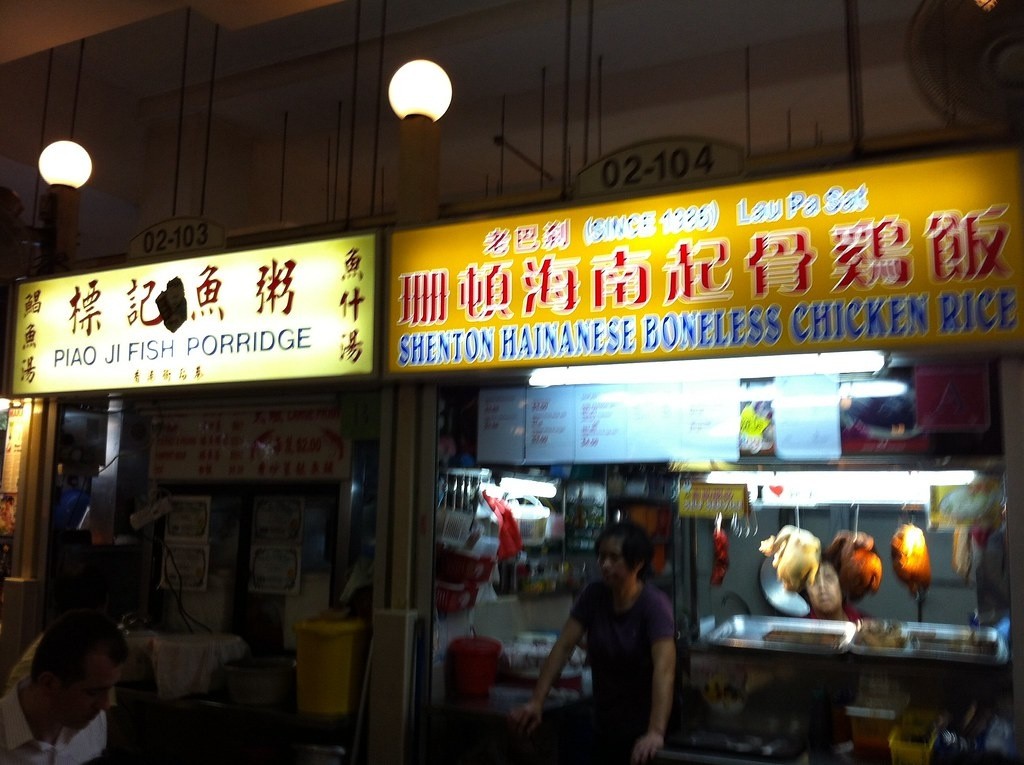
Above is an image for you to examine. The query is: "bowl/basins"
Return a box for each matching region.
[224,656,297,705]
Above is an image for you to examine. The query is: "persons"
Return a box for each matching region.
[798,554,874,623]
[508,520,675,765]
[0,564,131,765]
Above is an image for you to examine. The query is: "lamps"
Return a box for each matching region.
[38,140,93,191]
[387,59,453,122]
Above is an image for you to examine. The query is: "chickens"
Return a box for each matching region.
[759,524,933,623]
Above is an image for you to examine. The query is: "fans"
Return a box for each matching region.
[902,0,1024,139]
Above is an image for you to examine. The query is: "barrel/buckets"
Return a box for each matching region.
[450,625,502,693]
[292,616,365,716]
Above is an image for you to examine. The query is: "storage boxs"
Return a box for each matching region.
[887,724,938,765]
[903,708,944,729]
[849,717,902,759]
